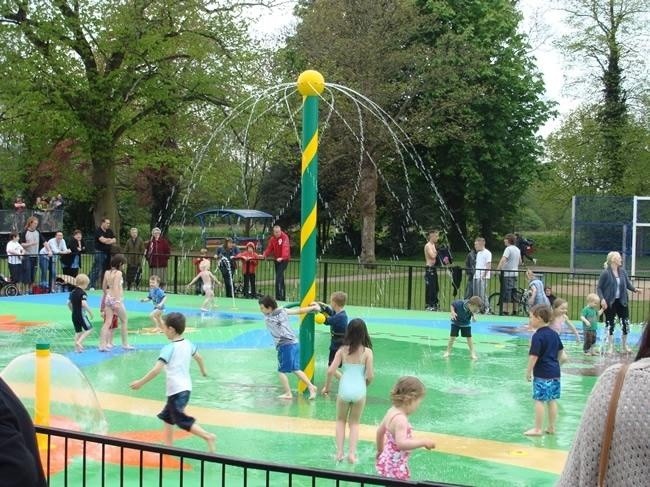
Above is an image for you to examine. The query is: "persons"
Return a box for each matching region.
[184,226,291,313]
[441,295,483,361]
[320,318,374,464]
[523,304,567,436]
[1,378,49,486]
[465,251,476,300]
[258,295,321,400]
[473,237,493,316]
[127,312,216,454]
[5,194,172,353]
[308,291,349,380]
[554,317,649,487]
[374,376,436,486]
[515,235,537,266]
[524,251,643,356]
[424,229,449,311]
[492,234,523,315]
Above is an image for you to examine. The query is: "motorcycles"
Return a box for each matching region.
[0,273,20,297]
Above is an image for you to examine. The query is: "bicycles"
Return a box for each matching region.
[232,276,264,300]
[489,285,530,316]
